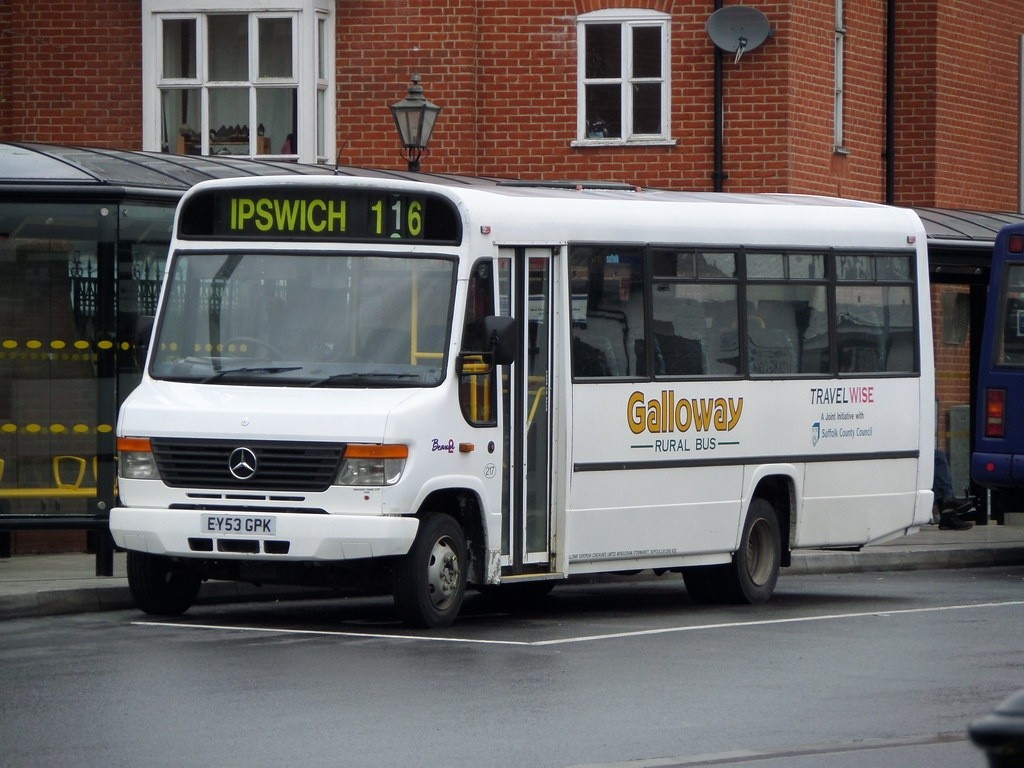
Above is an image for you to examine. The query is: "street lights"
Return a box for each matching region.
[386,70,444,175]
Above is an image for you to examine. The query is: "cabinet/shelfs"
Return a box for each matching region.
[177,134,272,156]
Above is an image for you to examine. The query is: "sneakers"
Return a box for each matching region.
[939,516,973,530]
[941,498,973,515]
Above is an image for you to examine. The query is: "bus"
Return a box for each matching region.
[109,174,940,632]
[969,224,1024,494]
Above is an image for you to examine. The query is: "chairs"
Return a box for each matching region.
[367,298,913,377]
